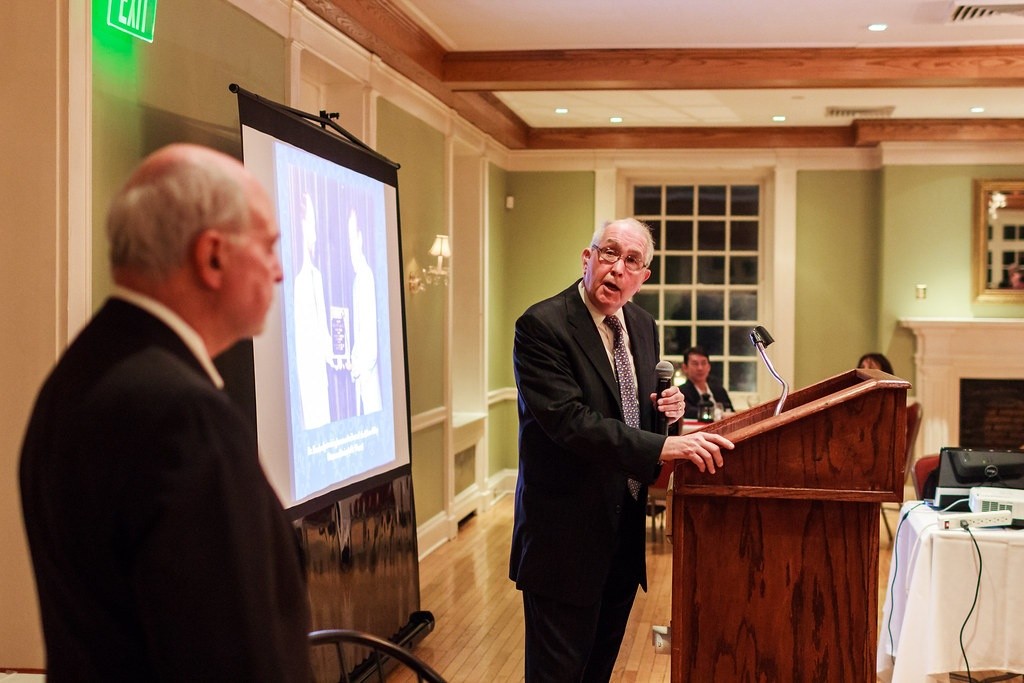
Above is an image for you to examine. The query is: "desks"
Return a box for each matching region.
[882,500,1024,683]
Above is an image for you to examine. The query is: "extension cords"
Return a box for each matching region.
[937,510,1013,530]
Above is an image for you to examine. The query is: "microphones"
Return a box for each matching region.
[655,360,674,435]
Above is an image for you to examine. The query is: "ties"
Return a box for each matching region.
[602,316,639,500]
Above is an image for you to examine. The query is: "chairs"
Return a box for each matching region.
[880,402,924,542]
[645,461,675,544]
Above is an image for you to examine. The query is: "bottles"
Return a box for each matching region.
[698,394,714,422]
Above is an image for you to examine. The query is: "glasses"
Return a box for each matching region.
[593,244,646,271]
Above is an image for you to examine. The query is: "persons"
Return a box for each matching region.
[288,189,385,428]
[507,218,735,683]
[17,138,313,683]
[672,348,736,430]
[857,352,896,376]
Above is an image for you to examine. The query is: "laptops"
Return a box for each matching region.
[924,447,1024,515]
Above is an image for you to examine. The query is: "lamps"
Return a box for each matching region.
[410,234,454,294]
